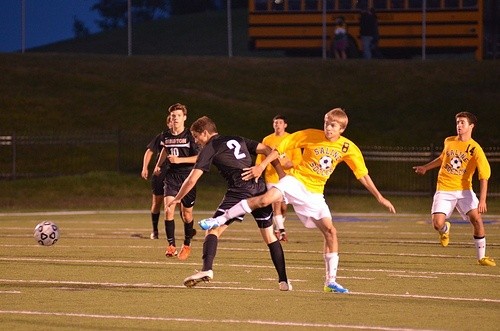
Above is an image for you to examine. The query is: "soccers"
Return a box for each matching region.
[33,221,59,246]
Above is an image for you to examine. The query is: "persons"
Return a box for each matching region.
[140,102,203,262]
[413,112,496,267]
[335,17,351,59]
[257,115,303,241]
[164,116,294,291]
[356,2,380,60]
[199,108,397,294]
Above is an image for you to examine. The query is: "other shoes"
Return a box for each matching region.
[150,232,158,239]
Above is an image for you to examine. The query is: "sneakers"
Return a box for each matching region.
[478,257,495,266]
[274,232,280,238]
[440,222,451,247]
[183,270,213,288]
[177,243,191,259]
[280,232,288,241]
[279,281,291,290]
[198,217,219,230]
[165,246,177,256]
[324,282,349,292]
